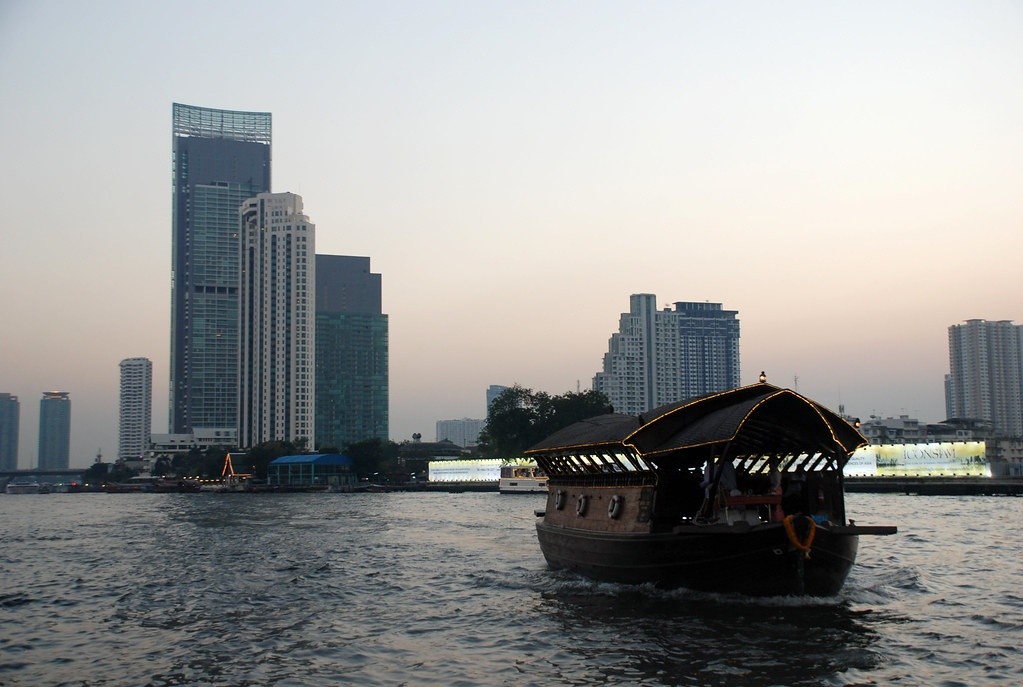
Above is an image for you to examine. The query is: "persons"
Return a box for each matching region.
[697,450,764,524]
[780,463,841,519]
[766,458,784,524]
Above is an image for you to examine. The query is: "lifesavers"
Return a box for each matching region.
[555,489,564,509]
[607,494,622,519]
[576,493,585,515]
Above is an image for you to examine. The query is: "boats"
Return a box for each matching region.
[524,370,898,605]
